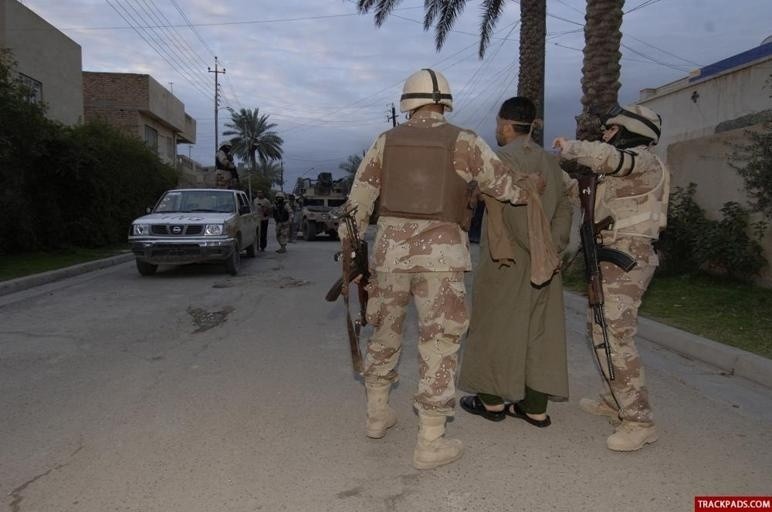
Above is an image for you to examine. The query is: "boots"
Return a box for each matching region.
[364,382,397,439]
[413,403,464,470]
[275,244,287,254]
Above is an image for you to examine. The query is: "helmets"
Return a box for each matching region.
[275,192,285,199]
[399,68,453,115]
[604,102,661,146]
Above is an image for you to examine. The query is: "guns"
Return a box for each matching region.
[578,173,637,381]
[326,199,400,388]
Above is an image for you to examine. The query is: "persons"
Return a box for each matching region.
[271,192,294,254]
[548,102,672,451]
[453,94,585,429]
[286,194,301,244]
[333,67,550,471]
[252,189,273,253]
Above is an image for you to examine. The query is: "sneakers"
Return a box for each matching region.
[607,419,658,452]
[579,397,617,419]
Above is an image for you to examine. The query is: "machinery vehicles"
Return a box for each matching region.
[294,169,347,241]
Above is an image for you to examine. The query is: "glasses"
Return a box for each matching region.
[605,105,622,118]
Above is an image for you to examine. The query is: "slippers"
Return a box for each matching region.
[505,401,551,428]
[459,395,505,422]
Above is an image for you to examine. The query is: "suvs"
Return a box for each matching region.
[119,185,263,276]
